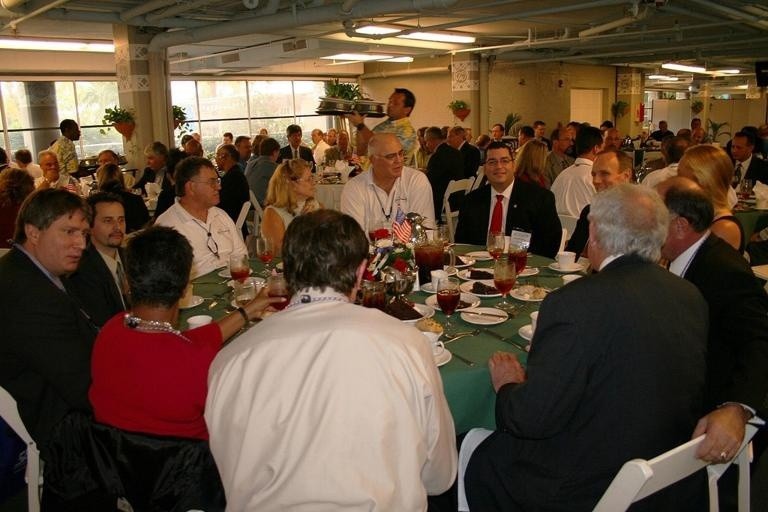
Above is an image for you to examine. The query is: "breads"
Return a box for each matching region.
[415,319,443,333]
[533,288,546,299]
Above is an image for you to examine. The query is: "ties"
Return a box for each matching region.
[115,261,134,314]
[294,149,297,160]
[735,163,742,182]
[488,194,505,241]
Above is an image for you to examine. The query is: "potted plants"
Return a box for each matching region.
[706,118,731,148]
[691,100,704,114]
[171,105,193,139]
[322,82,363,120]
[99,104,141,156]
[446,100,471,126]
[612,100,630,119]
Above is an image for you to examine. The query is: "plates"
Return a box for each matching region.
[392,248,547,366]
[218,267,253,278]
[319,95,386,105]
[178,295,206,311]
[732,194,758,212]
[548,263,586,273]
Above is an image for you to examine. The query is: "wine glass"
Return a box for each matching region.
[485,230,532,310]
[283,158,368,184]
[64,183,159,209]
[231,235,290,322]
[731,176,752,201]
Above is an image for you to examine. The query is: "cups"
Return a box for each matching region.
[187,314,213,328]
[179,282,193,303]
[415,222,457,288]
[358,283,387,311]
[557,249,576,269]
[561,275,582,287]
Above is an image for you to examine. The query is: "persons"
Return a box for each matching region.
[1,89,767,511]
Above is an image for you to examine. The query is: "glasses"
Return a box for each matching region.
[485,157,513,166]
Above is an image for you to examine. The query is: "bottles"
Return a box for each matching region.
[623,136,631,146]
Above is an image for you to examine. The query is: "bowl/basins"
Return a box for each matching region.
[79,159,97,167]
